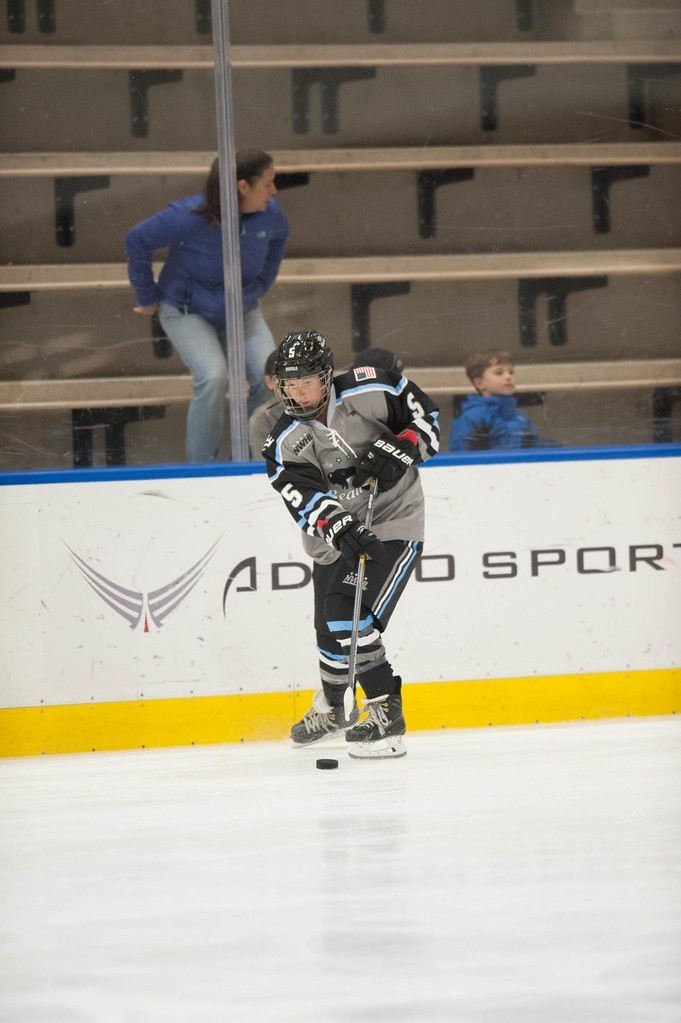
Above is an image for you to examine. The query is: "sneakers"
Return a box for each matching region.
[289,690,359,748]
[344,675,406,759]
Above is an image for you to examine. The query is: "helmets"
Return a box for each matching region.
[271,331,335,421]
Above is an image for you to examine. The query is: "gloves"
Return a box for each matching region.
[322,511,386,571]
[352,432,420,493]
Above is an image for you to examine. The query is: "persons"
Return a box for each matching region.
[126,147,290,463]
[262,330,440,757]
[448,346,559,451]
[250,349,285,462]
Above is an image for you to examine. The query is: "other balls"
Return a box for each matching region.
[316,759,339,769]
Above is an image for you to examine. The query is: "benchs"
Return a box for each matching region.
[0,1,681,473]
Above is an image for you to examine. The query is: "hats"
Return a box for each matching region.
[352,348,403,375]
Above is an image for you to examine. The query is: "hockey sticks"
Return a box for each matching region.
[344,477,378,722]
